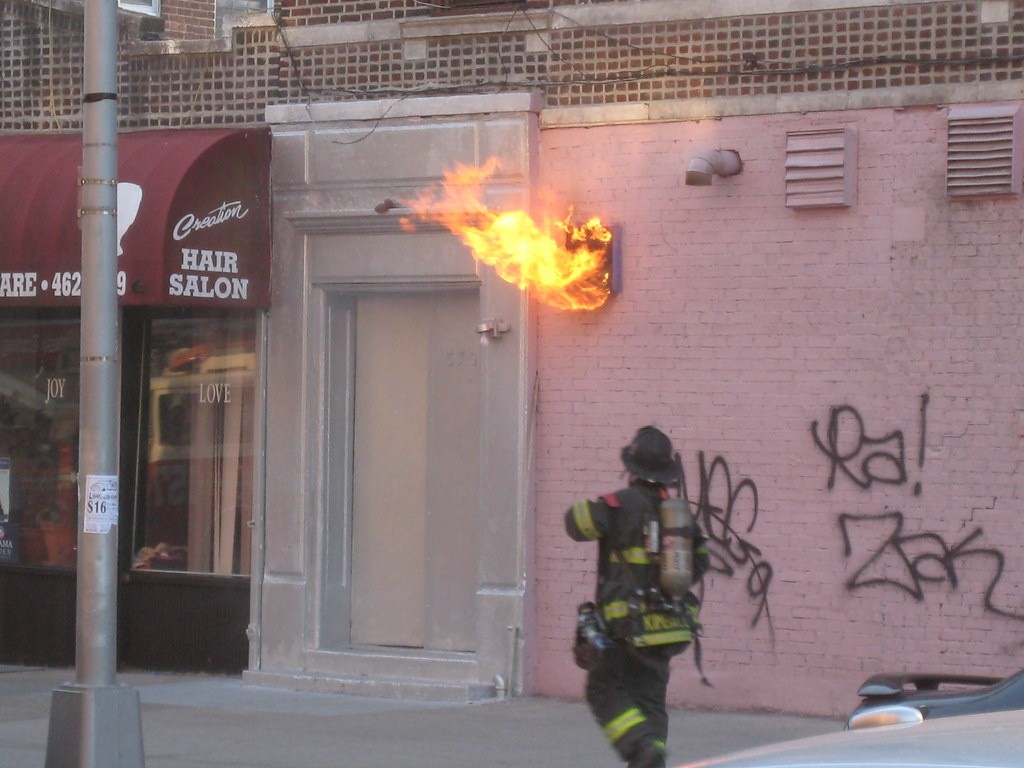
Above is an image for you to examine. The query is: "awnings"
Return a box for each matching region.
[0,123,271,314]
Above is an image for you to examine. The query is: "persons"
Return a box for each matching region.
[564,426,712,768]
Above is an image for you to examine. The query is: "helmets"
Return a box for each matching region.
[622,426,679,483]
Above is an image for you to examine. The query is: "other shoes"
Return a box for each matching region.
[627,738,665,768]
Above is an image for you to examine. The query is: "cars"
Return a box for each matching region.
[674,708,1023,768]
[840,660,1024,730]
